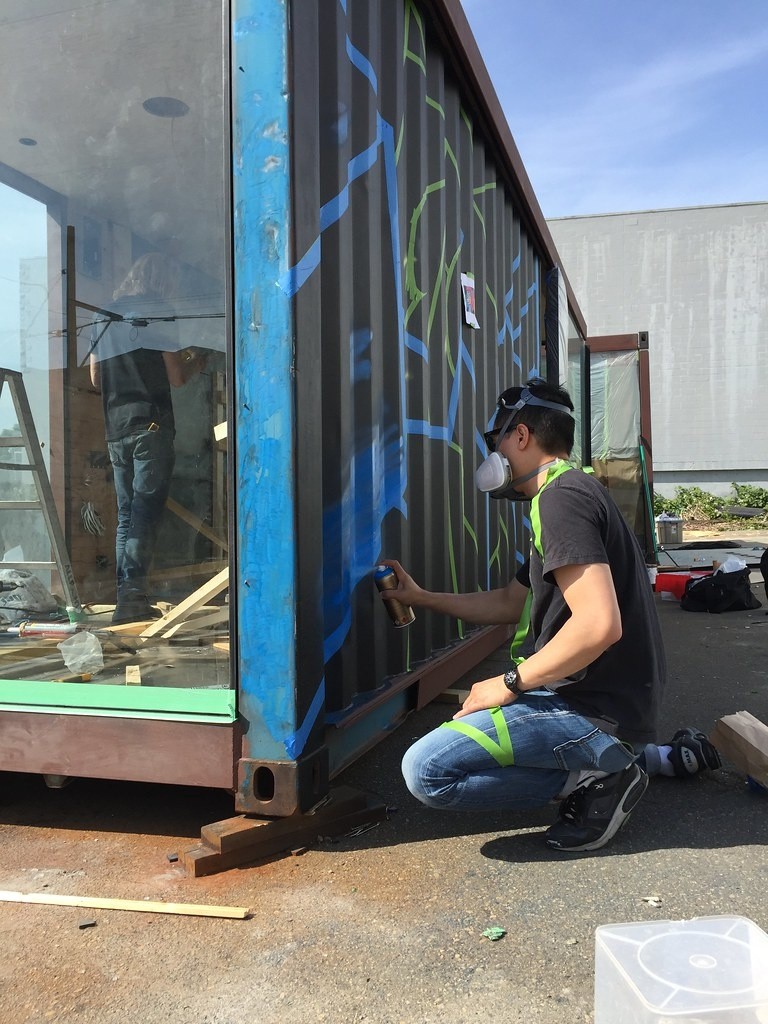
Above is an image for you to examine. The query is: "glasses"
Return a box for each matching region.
[482,425,535,450]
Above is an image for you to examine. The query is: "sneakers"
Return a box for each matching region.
[112,600,162,624]
[544,766,648,851]
[665,725,722,779]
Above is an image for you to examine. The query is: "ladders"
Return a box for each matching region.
[0,369,92,628]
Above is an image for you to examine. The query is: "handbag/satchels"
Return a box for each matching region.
[677,567,762,612]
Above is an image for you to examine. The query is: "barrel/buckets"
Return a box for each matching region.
[656,518,684,544]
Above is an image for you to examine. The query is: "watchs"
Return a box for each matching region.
[500,667,528,696]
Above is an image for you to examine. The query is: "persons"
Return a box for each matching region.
[84,249,210,623]
[375,376,722,854]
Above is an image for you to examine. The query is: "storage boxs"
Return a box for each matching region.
[657,569,714,601]
[656,520,685,544]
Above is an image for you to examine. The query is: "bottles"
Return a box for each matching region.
[375,566,417,628]
